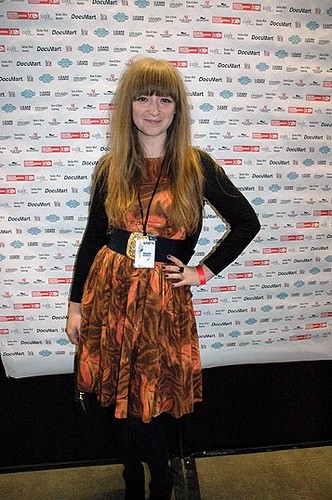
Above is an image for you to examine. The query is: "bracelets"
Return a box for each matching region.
[195,265,206,285]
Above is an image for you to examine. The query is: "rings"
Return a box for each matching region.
[178,266,184,273]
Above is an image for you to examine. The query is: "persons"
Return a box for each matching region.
[66,58,262,499]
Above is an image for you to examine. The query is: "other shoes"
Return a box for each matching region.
[122,463,175,500]
